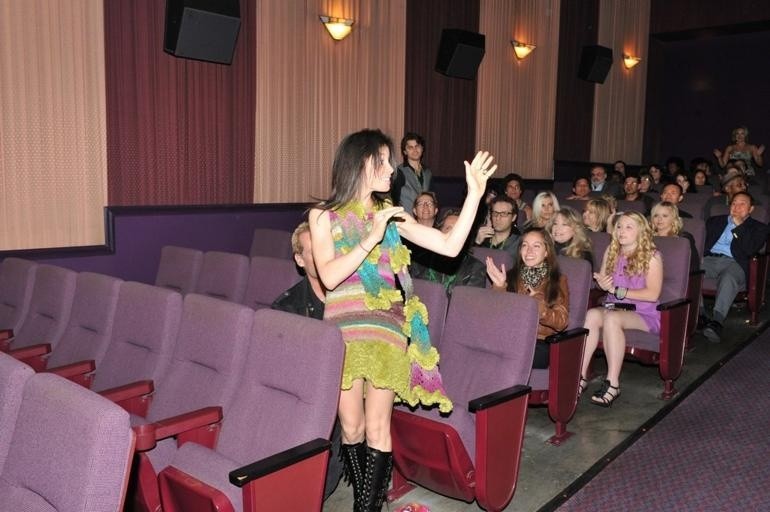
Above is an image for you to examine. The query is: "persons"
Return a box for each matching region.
[693,171,713,196]
[483,225,571,371]
[712,124,765,176]
[467,193,520,286]
[638,171,662,205]
[702,170,763,223]
[581,195,618,238]
[613,160,629,183]
[566,176,594,208]
[576,209,664,406]
[501,175,533,224]
[547,207,599,273]
[696,193,764,344]
[523,188,562,231]
[416,207,488,291]
[658,181,695,222]
[650,200,684,241]
[308,128,499,511]
[614,172,655,216]
[402,190,440,281]
[588,163,620,196]
[667,161,752,181]
[674,172,693,198]
[609,170,625,190]
[391,133,441,223]
[483,172,499,220]
[648,164,666,189]
[269,219,326,322]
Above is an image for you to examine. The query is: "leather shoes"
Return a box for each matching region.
[703,326,720,343]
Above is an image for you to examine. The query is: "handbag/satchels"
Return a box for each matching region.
[604,302,637,312]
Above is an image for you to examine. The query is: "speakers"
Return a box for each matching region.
[577,45,613,84]
[434,28,486,81]
[162,1,242,66]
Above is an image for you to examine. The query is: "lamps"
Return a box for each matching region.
[623,56,642,69]
[510,41,537,59]
[319,16,355,41]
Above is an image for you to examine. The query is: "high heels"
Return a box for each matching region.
[590,379,621,408]
[578,375,587,396]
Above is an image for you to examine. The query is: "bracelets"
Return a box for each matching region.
[614,285,627,296]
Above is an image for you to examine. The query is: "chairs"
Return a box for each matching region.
[0,258,36,349]
[10,265,77,371]
[249,229,291,260]
[597,236,693,400]
[45,271,122,387]
[429,175,769,292]
[155,246,202,300]
[390,286,538,510]
[196,251,249,304]
[87,280,181,416]
[243,258,304,308]
[0,352,35,511]
[136,309,346,512]
[411,279,448,350]
[95,294,253,512]
[1,373,136,512]
[528,257,592,446]
[702,205,770,325]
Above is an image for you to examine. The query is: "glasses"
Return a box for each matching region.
[625,181,638,185]
[491,210,514,217]
[415,200,436,207]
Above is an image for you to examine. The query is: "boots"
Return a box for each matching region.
[341,440,366,510]
[359,445,393,512]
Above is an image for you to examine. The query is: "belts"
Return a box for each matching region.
[707,251,724,257]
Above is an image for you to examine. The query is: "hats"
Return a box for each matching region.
[721,172,741,191]
